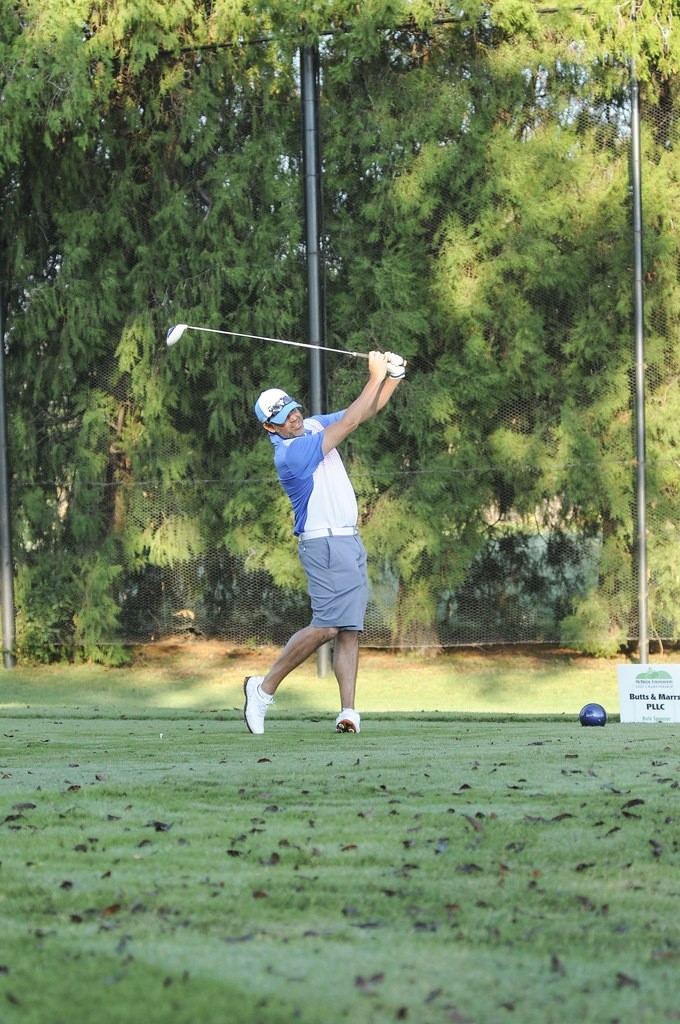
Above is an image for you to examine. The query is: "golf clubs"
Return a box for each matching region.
[165,323,408,367]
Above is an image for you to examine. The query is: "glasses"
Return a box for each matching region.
[266,396,294,422]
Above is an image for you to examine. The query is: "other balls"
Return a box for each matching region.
[579,703,606,726]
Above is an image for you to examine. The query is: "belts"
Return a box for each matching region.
[298,526,359,542]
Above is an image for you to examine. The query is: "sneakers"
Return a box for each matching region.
[243,675,274,734]
[335,709,360,733]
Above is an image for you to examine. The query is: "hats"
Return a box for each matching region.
[255,388,303,425]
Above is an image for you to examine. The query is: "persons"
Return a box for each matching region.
[244,350,403,732]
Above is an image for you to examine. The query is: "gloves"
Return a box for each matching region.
[384,351,406,380]
